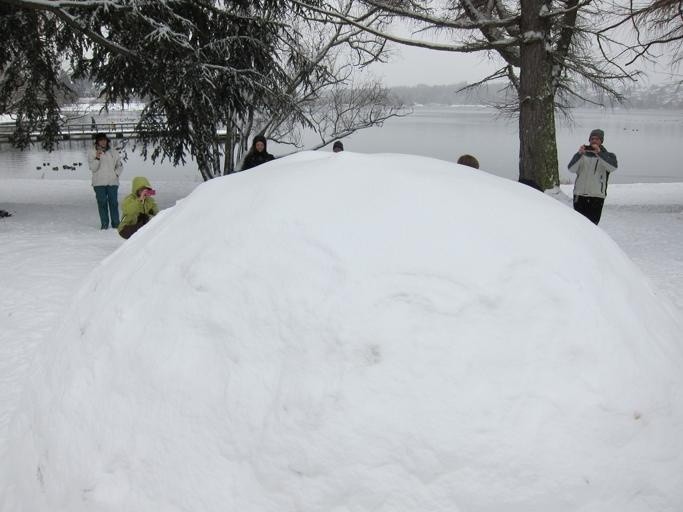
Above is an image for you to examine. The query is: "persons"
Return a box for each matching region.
[111,122,116,132]
[458,154,479,169]
[568,128,617,225]
[91,117,96,131]
[241,134,275,171]
[333,140,343,152]
[89,133,123,229]
[118,177,160,239]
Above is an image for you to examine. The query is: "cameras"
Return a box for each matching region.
[584,145,593,150]
[146,189,155,196]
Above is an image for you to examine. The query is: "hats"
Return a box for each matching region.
[95,133,110,144]
[589,129,604,143]
